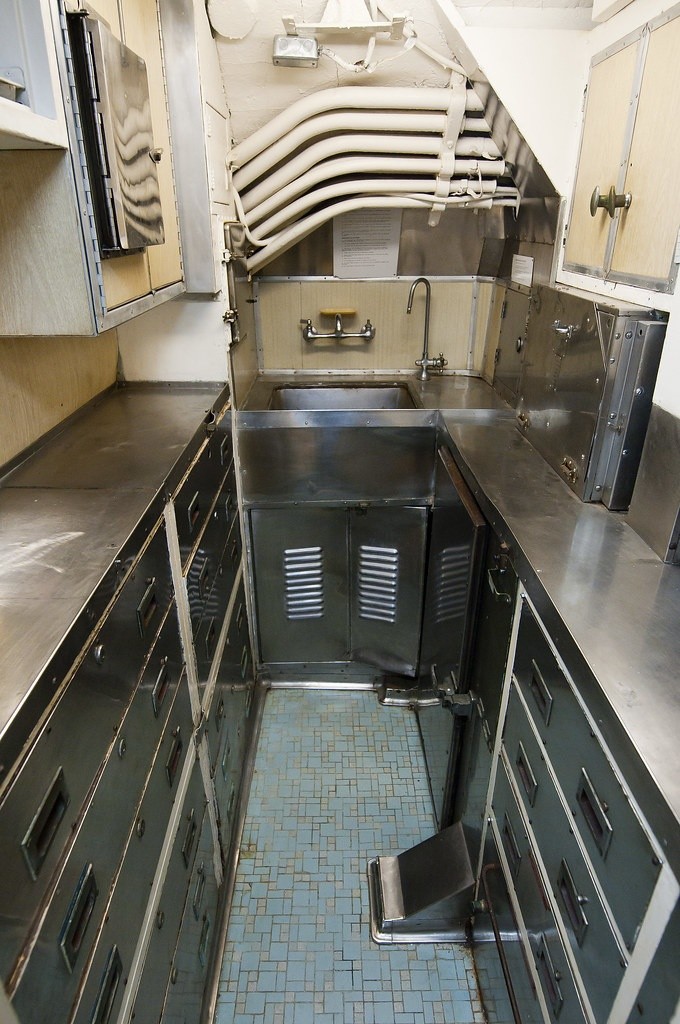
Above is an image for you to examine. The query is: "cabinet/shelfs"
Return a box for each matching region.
[1,2,680,1024]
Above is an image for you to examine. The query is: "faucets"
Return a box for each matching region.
[405,278,449,382]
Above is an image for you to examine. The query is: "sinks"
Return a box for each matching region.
[264,378,427,414]
